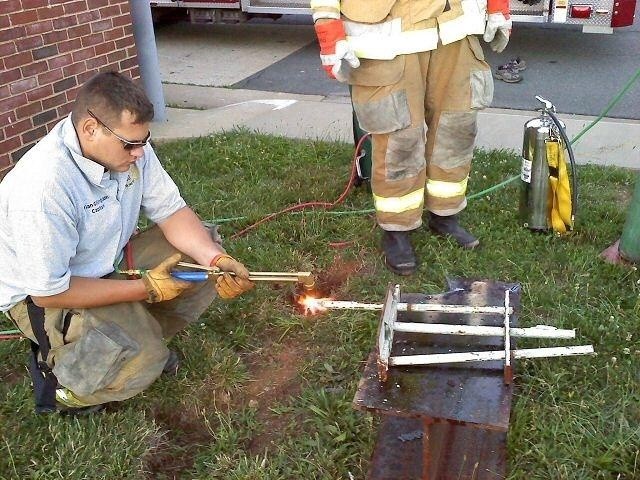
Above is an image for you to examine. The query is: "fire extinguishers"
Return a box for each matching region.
[519,95,578,232]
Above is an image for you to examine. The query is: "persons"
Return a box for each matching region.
[311,0,514,275]
[0,71,252,417]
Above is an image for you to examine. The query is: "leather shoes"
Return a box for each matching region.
[382,230,417,276]
[428,213,478,249]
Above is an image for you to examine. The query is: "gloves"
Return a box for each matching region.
[210,255,253,300]
[484,0,514,55]
[144,254,194,304]
[315,19,359,82]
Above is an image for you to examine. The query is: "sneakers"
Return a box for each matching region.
[509,58,527,71]
[60,403,103,417]
[493,65,523,83]
[165,344,187,375]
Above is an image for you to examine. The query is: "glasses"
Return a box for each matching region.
[87,109,152,149]
